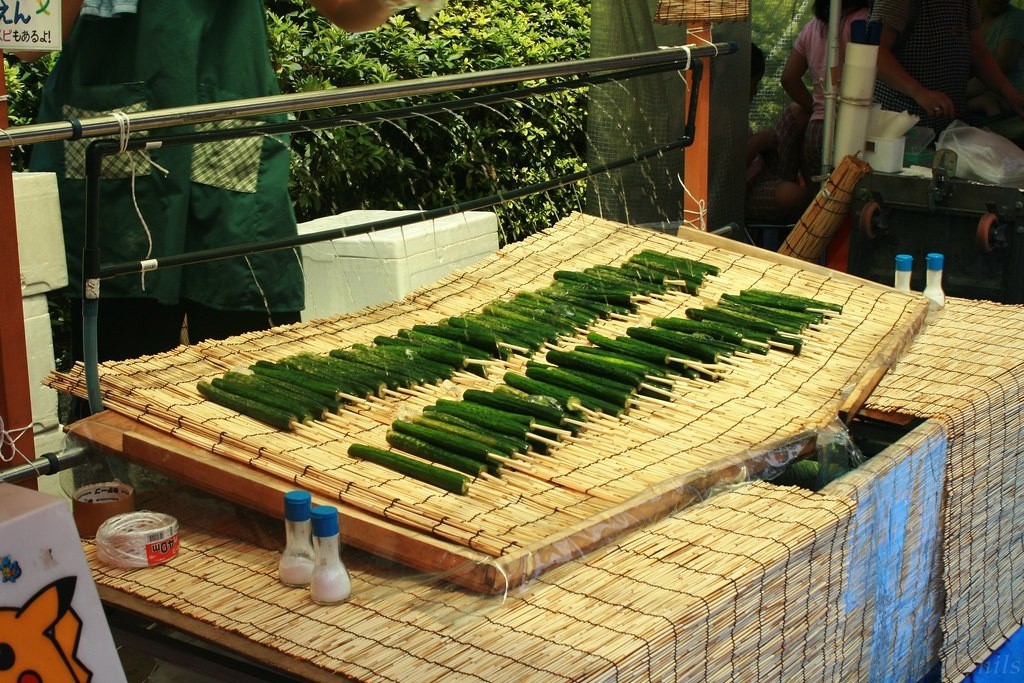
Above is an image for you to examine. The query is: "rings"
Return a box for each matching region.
[934,107,940,111]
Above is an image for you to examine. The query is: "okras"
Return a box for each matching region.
[197,247,846,494]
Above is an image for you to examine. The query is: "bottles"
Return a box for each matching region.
[309,507,351,606]
[922,253,945,310]
[279,490,317,588]
[893,254,913,290]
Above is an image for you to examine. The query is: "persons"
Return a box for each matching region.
[867,0,1024,167]
[781,0,881,198]
[1,0,436,497]
[747,42,809,214]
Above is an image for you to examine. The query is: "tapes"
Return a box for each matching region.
[72,481,135,540]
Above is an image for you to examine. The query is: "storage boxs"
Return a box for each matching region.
[12,173,68,495]
[296,210,499,323]
[864,135,906,173]
